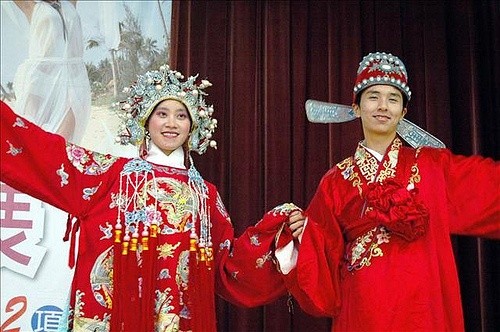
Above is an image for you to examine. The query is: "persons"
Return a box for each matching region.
[1,65,303,332]
[290,52,500,332]
[1,0,93,142]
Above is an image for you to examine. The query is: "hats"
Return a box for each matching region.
[305,52,446,149]
[110,65,217,332]
